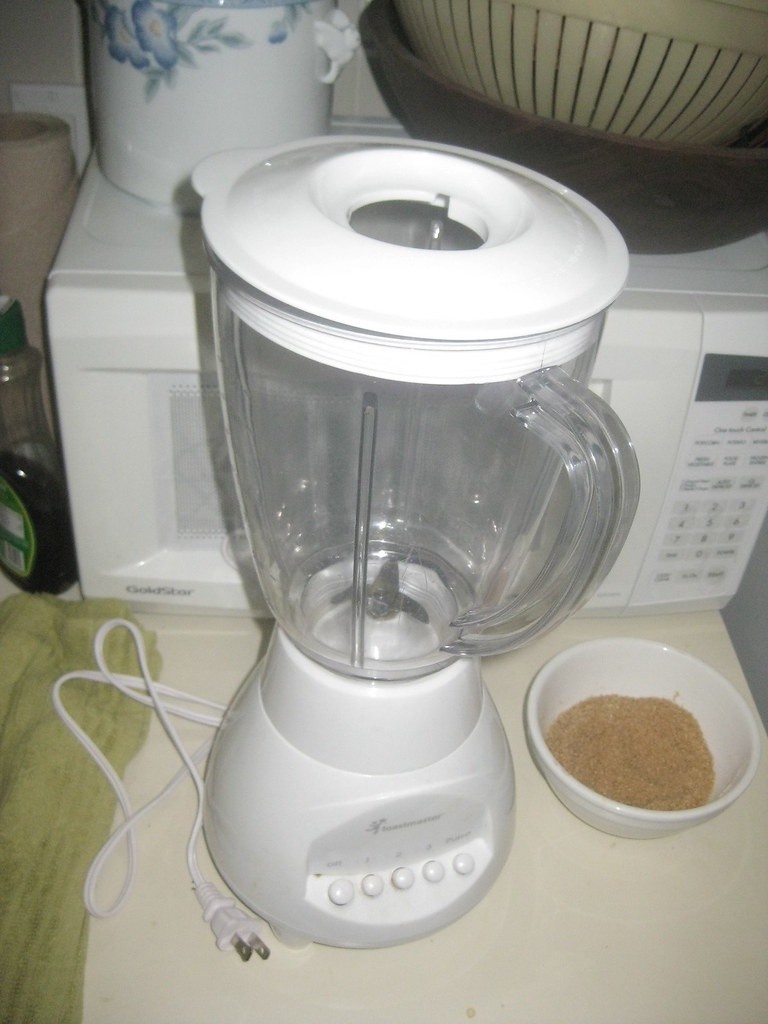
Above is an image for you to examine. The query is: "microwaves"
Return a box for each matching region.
[41,141,768,618]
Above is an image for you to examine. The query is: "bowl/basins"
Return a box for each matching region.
[523,637,761,841]
[393,0,768,146]
[352,0,768,254]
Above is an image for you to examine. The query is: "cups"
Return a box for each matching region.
[79,0,363,207]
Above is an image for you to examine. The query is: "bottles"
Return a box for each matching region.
[0,294,79,597]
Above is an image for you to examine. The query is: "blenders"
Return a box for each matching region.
[190,134,643,950]
